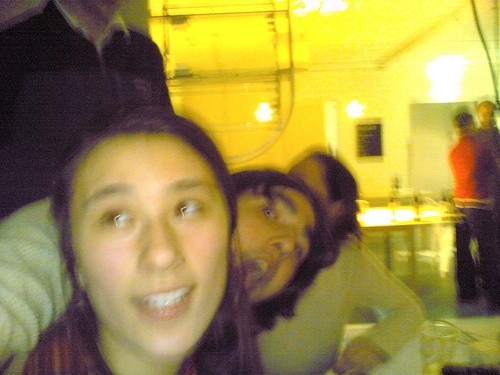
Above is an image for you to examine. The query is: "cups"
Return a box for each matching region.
[420,325,460,375]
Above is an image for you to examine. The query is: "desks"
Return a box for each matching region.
[356,202,465,278]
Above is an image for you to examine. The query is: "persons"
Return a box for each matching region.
[243,151,428,375]
[4,109,266,375]
[0,1,175,226]
[468,100,499,180]
[1,168,336,367]
[447,112,499,303]
